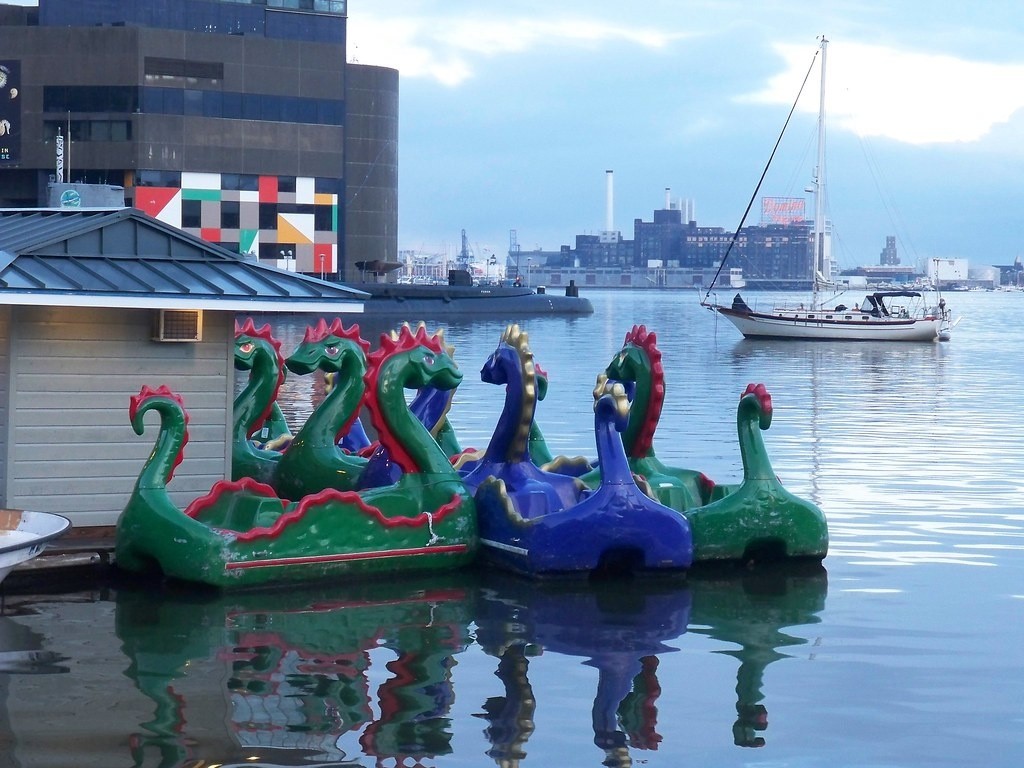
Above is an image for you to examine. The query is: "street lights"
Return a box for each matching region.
[318,253,326,280]
[527,257,534,288]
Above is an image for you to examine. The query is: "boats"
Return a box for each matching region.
[0,509,73,585]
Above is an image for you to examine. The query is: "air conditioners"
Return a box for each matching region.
[151,309,203,342]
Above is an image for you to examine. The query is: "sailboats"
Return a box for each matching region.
[701,35,963,341]
